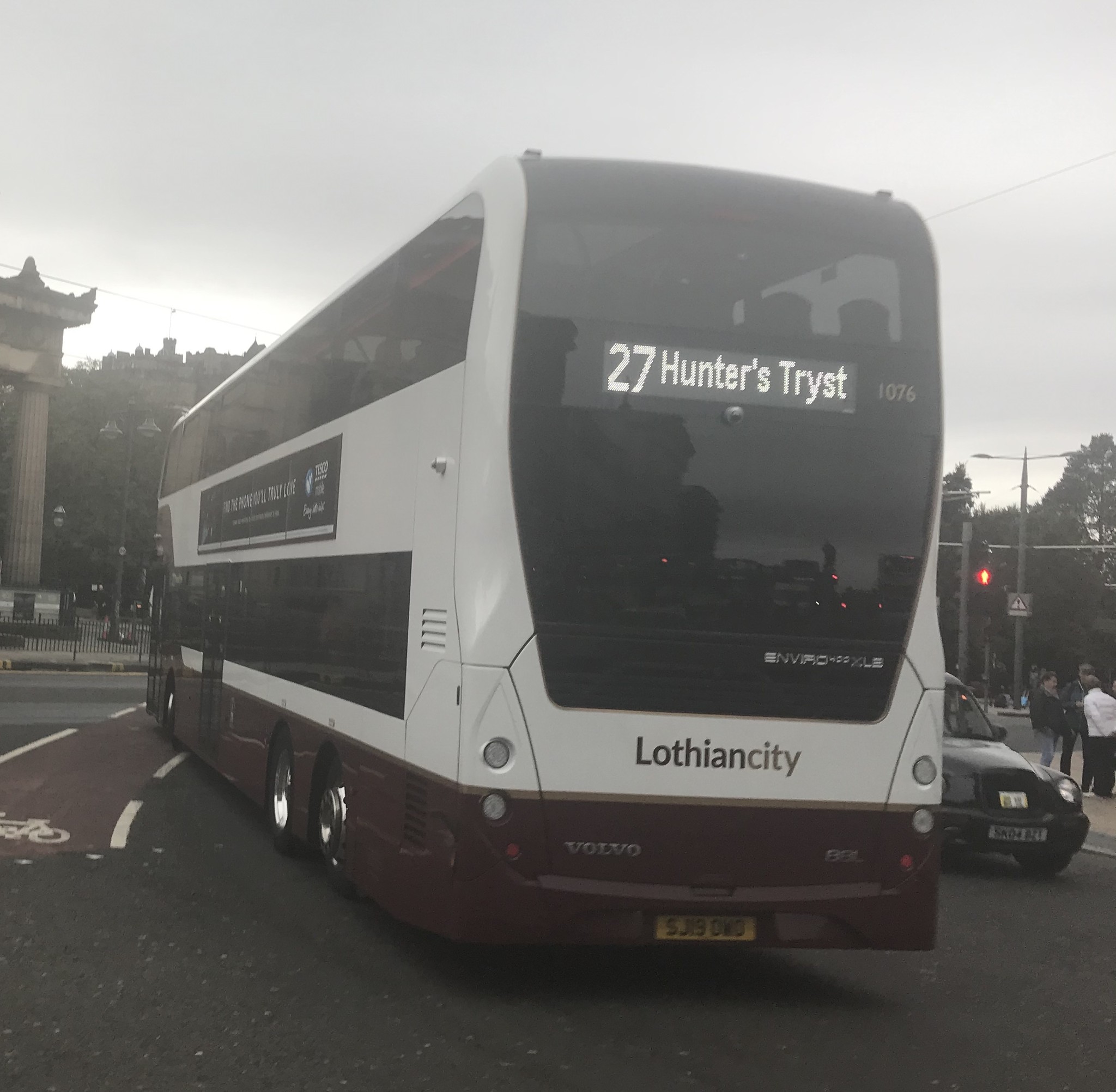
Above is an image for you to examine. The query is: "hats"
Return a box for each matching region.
[1078,664,1095,677]
[1080,675,1100,687]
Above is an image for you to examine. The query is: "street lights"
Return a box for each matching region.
[50,504,66,590]
[98,403,161,643]
[973,448,1082,710]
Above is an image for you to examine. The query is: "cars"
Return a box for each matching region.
[939,672,1091,875]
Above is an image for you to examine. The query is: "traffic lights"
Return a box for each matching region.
[972,539,994,612]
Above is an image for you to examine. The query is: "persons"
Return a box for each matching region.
[979,653,1047,709]
[1029,664,1116,777]
[1082,675,1116,797]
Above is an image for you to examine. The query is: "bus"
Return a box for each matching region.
[145,150,944,952]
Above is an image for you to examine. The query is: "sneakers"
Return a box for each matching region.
[1082,791,1095,797]
